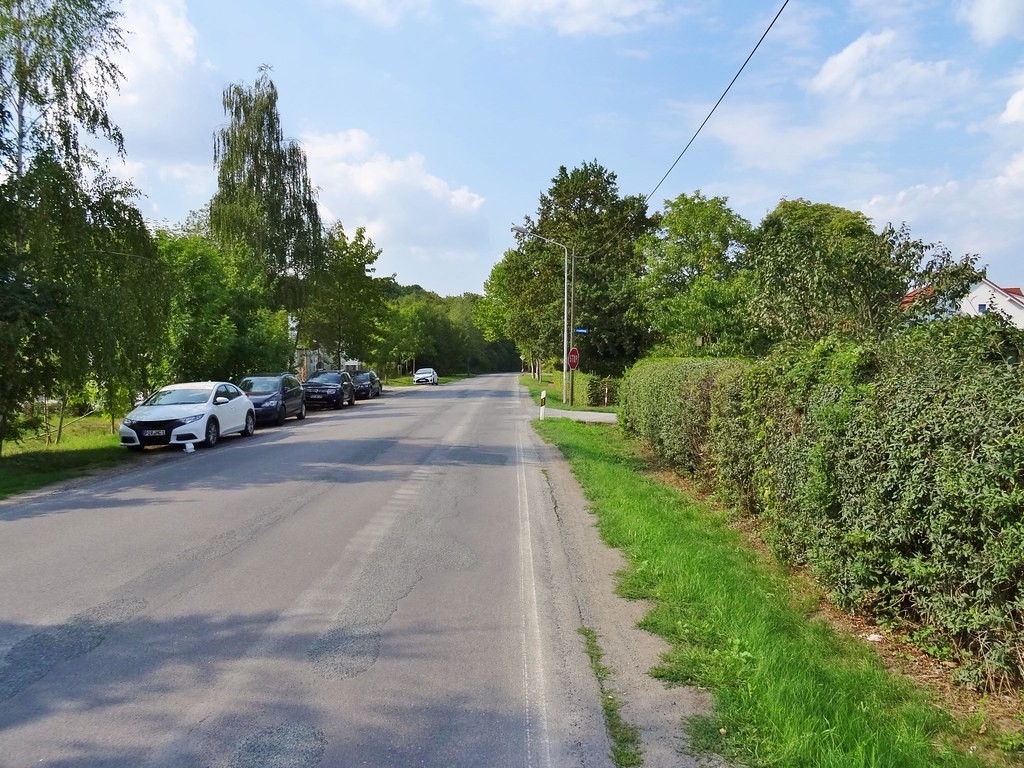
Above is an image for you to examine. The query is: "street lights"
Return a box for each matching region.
[509,224,568,405]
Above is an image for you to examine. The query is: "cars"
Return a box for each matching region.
[413,368,439,385]
[343,370,382,399]
[118,381,256,448]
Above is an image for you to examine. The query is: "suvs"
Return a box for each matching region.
[300,368,355,410]
[236,372,307,426]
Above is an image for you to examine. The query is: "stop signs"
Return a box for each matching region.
[568,348,580,371]
